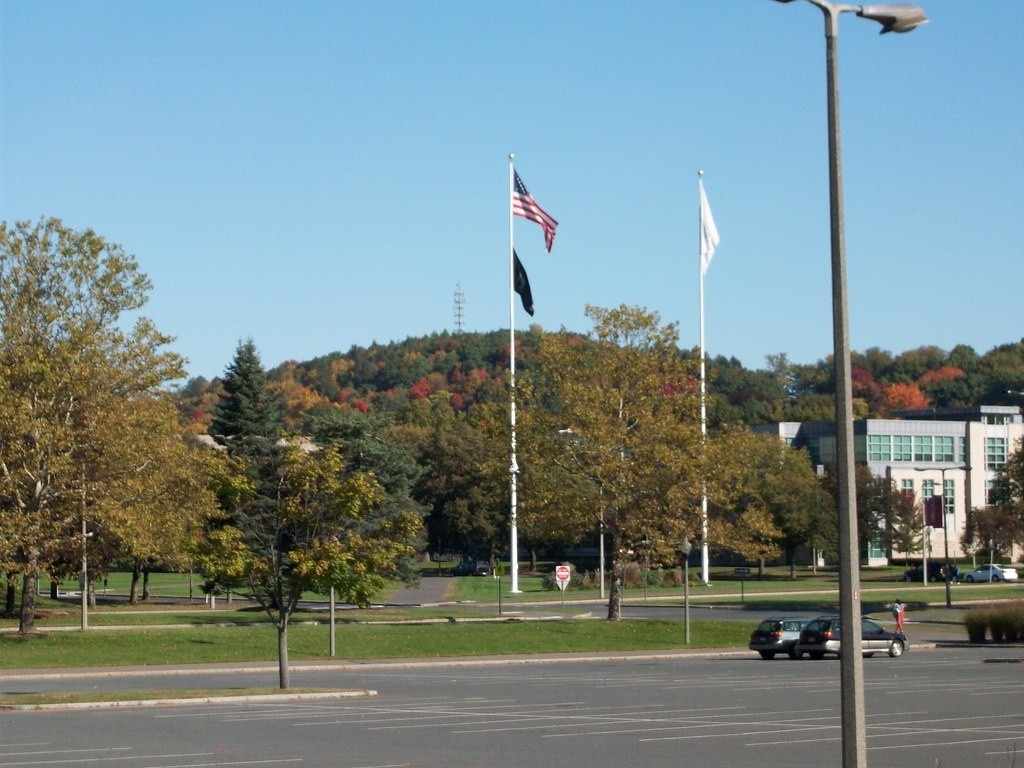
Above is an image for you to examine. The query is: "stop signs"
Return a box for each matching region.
[556,566,570,582]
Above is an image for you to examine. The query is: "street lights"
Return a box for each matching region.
[557,426,607,599]
[780,0,933,767]
[913,463,973,607]
[680,536,693,645]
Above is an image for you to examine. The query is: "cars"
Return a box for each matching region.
[796,615,911,661]
[448,558,492,577]
[962,562,1018,584]
[747,616,818,660]
[903,561,958,582]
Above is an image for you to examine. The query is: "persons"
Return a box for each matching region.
[893,598,904,635]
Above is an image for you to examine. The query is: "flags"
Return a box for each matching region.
[513,170,558,252]
[699,181,720,277]
[512,249,534,315]
